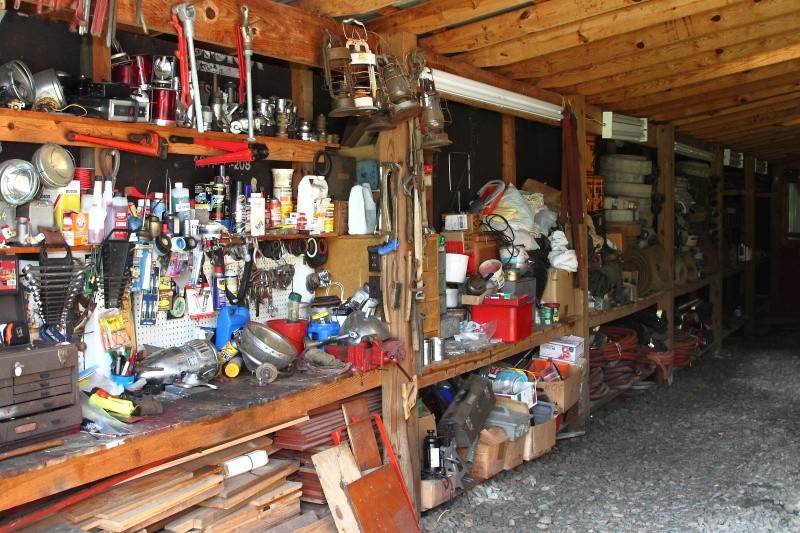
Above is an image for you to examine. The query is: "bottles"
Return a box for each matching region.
[349,282,370,311]
[15,217,30,243]
[185,267,250,378]
[308,320,341,352]
[61,174,379,246]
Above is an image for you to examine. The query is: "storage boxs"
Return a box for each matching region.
[43,182,80,246]
[421,211,586,511]
[607,230,629,252]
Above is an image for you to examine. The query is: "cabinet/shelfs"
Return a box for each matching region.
[0,108,339,256]
[421,237,440,340]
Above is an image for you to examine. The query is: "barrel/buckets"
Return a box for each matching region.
[445,289,459,307]
[445,253,470,283]
[478,259,506,289]
[267,319,308,358]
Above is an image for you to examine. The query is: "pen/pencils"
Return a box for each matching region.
[111,350,138,375]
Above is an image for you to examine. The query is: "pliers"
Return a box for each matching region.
[252,238,265,263]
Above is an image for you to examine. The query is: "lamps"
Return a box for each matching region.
[0,59,76,207]
[724,149,744,169]
[233,321,296,387]
[675,142,716,162]
[601,111,649,144]
[755,157,768,174]
[322,18,564,149]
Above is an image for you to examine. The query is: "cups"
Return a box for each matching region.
[505,267,560,325]
[423,336,445,366]
[111,372,134,390]
[271,168,294,187]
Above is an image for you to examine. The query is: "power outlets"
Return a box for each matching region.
[369,251,381,272]
[369,276,381,298]
